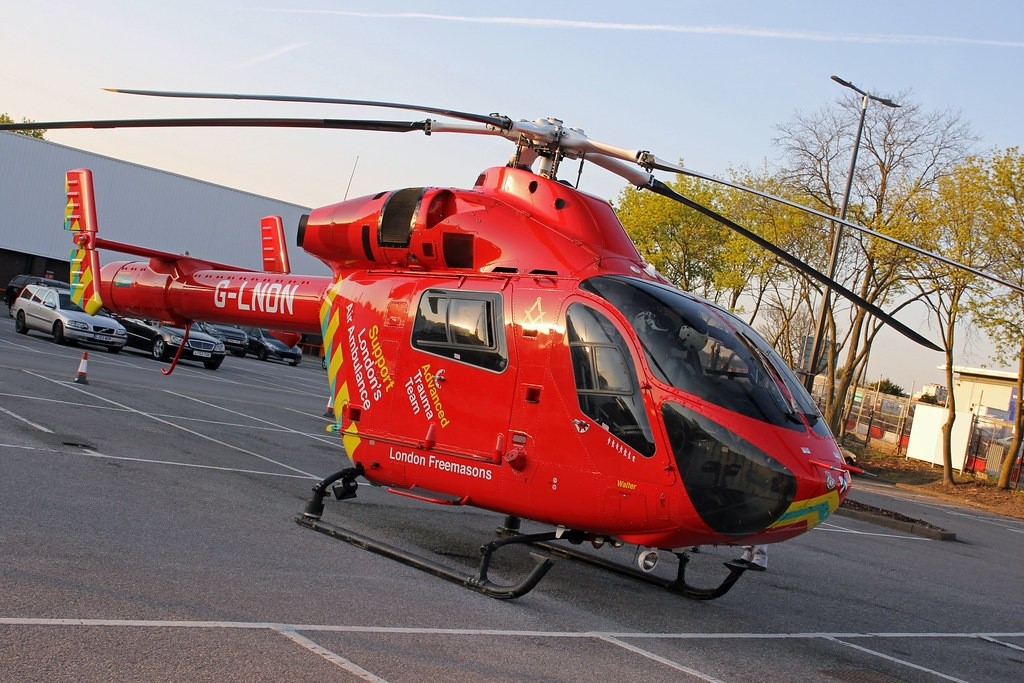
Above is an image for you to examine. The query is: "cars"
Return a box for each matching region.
[190,321,249,358]
[241,326,303,367]
[5,274,128,354]
[114,314,226,371]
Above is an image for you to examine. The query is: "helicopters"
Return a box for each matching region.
[0,88,1024,600]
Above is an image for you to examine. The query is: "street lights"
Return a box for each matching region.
[804,75,902,395]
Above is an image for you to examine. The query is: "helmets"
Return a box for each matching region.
[671,314,709,352]
